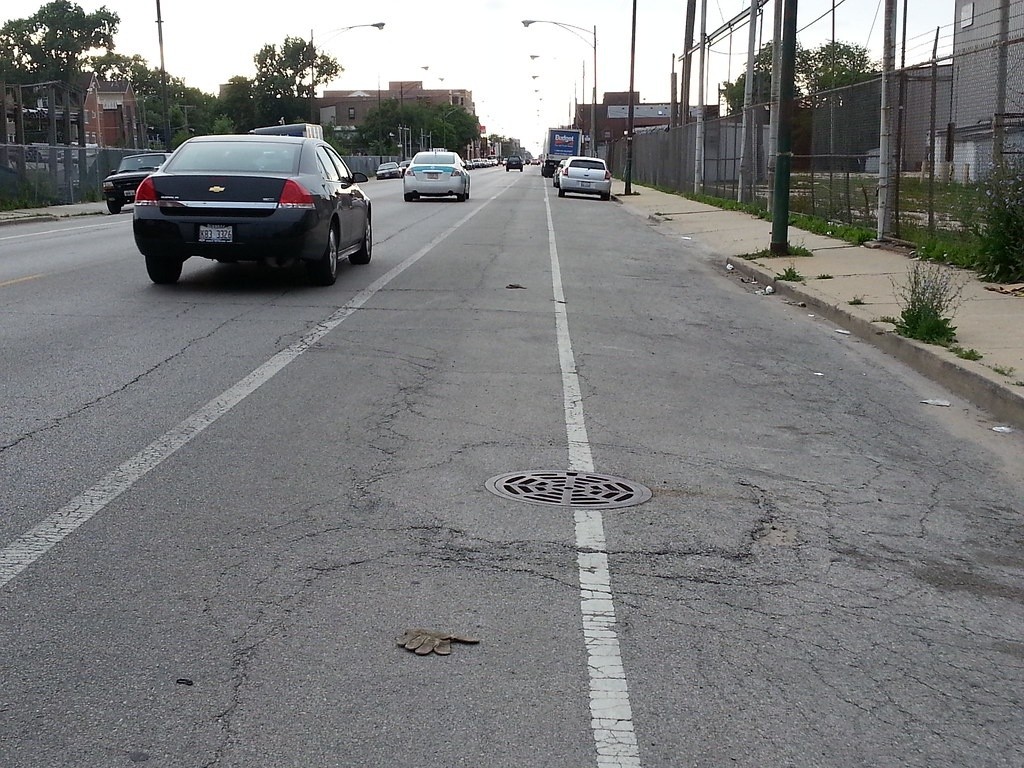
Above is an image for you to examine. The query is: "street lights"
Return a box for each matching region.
[310,22,485,152]
[520,20,596,159]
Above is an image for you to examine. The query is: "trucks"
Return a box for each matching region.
[249,116,323,141]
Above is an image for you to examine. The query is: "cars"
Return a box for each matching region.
[553,160,567,188]
[557,156,612,201]
[101,153,171,214]
[134,135,373,287]
[376,158,498,179]
[531,158,539,166]
[503,157,509,167]
[402,151,470,203]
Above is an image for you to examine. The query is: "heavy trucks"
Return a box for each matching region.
[540,127,583,178]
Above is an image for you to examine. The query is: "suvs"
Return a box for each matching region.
[506,155,523,172]
